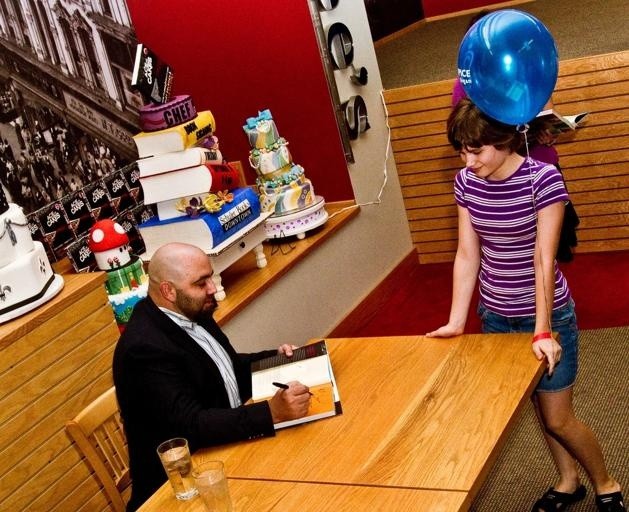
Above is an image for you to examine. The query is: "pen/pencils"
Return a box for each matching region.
[272,382,314,395]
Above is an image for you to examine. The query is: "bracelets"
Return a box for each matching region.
[532,331,551,343]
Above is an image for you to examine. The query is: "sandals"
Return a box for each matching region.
[530,485,587,512]
[595,492,626,512]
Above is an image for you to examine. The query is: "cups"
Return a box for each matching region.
[156,438,198,499]
[192,460,233,512]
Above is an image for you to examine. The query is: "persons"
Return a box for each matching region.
[112,242,310,512]
[0,119,122,203]
[426,98,626,512]
[453,9,579,263]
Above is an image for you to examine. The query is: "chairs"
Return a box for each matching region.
[63,385,134,512]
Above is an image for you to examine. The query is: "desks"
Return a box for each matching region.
[135,332,560,512]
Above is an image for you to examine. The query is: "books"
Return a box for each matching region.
[533,109,589,134]
[250,340,343,429]
[131,44,175,104]
[132,110,260,256]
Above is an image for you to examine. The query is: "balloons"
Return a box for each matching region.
[456,9,558,125]
